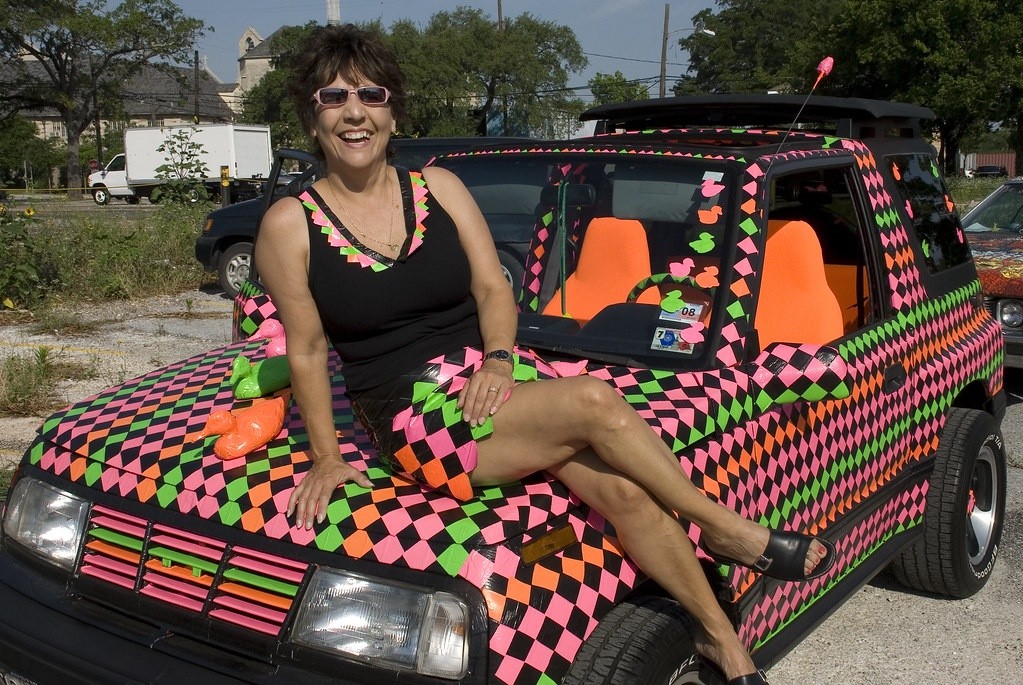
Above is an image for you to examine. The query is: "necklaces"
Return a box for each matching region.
[325,174,400,252]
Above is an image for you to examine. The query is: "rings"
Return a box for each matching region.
[488,387,498,393]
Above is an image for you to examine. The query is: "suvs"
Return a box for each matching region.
[0,95,1011,685]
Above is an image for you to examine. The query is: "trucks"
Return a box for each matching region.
[87,126,273,206]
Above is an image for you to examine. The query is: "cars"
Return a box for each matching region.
[288,171,315,185]
[962,170,974,179]
[195,138,550,302]
[974,165,1008,177]
[274,167,308,196]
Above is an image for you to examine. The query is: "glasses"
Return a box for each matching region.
[310,86,393,107]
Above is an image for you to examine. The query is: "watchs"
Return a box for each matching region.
[483,350,514,371]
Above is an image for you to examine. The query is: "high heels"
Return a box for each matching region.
[698,657,770,685]
[703,521,836,580]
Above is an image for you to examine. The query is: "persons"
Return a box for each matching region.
[254,23,837,685]
